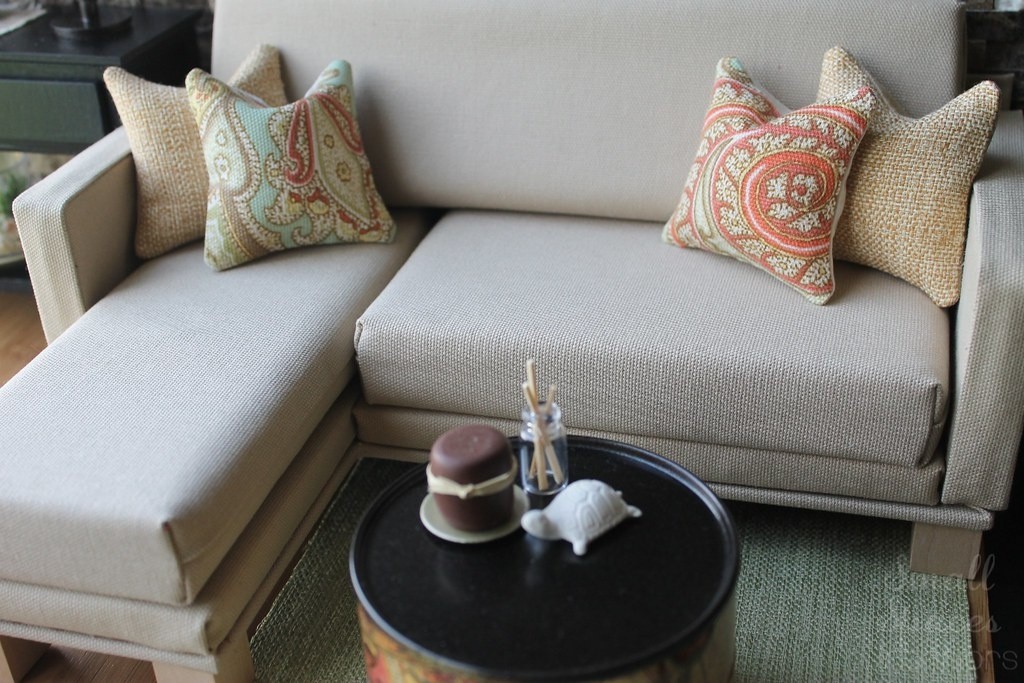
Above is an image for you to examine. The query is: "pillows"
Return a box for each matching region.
[663,55,875,302]
[816,46,1000,308]
[101,46,290,258]
[183,58,395,273]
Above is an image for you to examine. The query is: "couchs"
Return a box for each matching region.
[0,0,1024,683]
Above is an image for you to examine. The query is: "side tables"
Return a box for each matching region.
[0,9,202,303]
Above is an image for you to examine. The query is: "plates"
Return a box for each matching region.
[420,483,530,545]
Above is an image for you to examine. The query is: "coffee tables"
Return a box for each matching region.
[348,435,739,683]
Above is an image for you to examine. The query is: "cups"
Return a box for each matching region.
[517,401,570,495]
